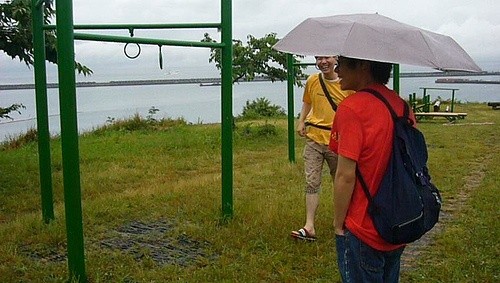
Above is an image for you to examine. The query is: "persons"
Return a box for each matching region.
[290,55,356,240]
[328,56,416,283]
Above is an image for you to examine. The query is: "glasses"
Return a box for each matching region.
[335,58,353,64]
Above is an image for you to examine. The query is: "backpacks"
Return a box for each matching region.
[354,89,443,244]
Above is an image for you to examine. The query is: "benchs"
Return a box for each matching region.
[488,102,500,110]
[414,112,467,123]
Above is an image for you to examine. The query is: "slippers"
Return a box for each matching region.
[290,227,318,242]
